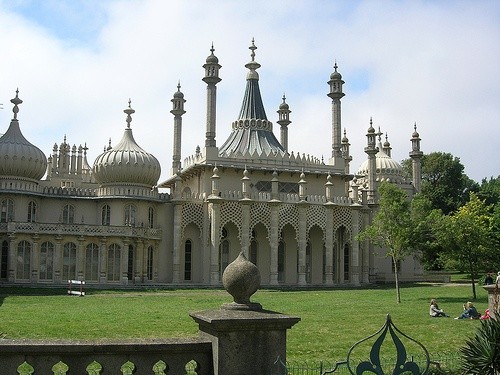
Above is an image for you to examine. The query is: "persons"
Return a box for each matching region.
[429,299,450,317]
[454,302,479,320]
